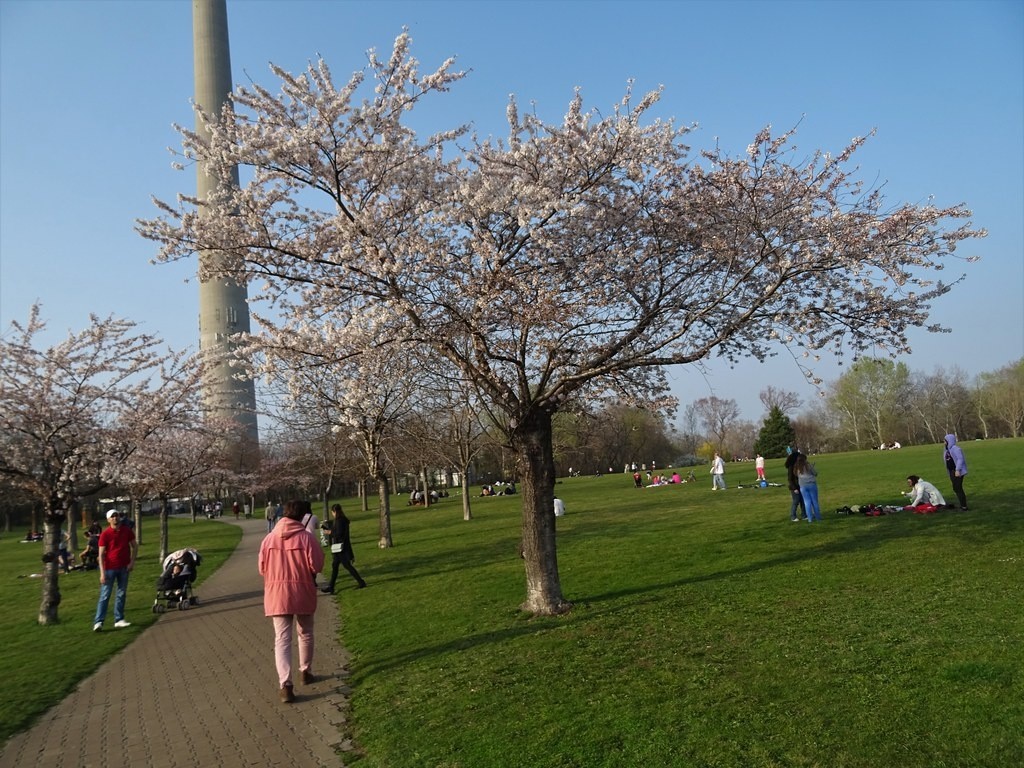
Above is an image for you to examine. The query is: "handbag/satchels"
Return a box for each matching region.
[330,543,344,553]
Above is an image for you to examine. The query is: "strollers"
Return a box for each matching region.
[152,551,200,613]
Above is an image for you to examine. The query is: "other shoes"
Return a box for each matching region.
[300,670,314,685]
[65,571,72,574]
[355,580,367,589]
[959,506,968,512]
[711,486,718,491]
[280,685,296,703]
[791,518,799,522]
[321,587,335,595]
[756,478,761,482]
[762,477,766,481]
[802,518,808,520]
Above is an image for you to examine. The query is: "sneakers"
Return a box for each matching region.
[114,619,132,628]
[93,622,103,632]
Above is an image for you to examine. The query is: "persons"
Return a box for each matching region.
[244,503,250,519]
[321,504,366,593]
[901,476,946,514]
[410,489,449,502]
[756,453,766,481]
[944,434,970,512]
[712,453,727,490]
[26,529,44,542]
[198,499,223,519]
[168,565,181,579]
[258,501,325,702]
[59,512,135,574]
[94,509,132,631]
[265,502,276,532]
[645,470,681,485]
[785,452,808,521]
[873,441,901,450]
[276,503,283,521]
[233,502,239,519]
[624,460,656,473]
[794,454,823,522]
[482,480,516,497]
[554,496,565,516]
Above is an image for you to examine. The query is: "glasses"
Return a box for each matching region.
[111,514,118,517]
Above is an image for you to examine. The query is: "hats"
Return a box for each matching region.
[106,508,119,519]
